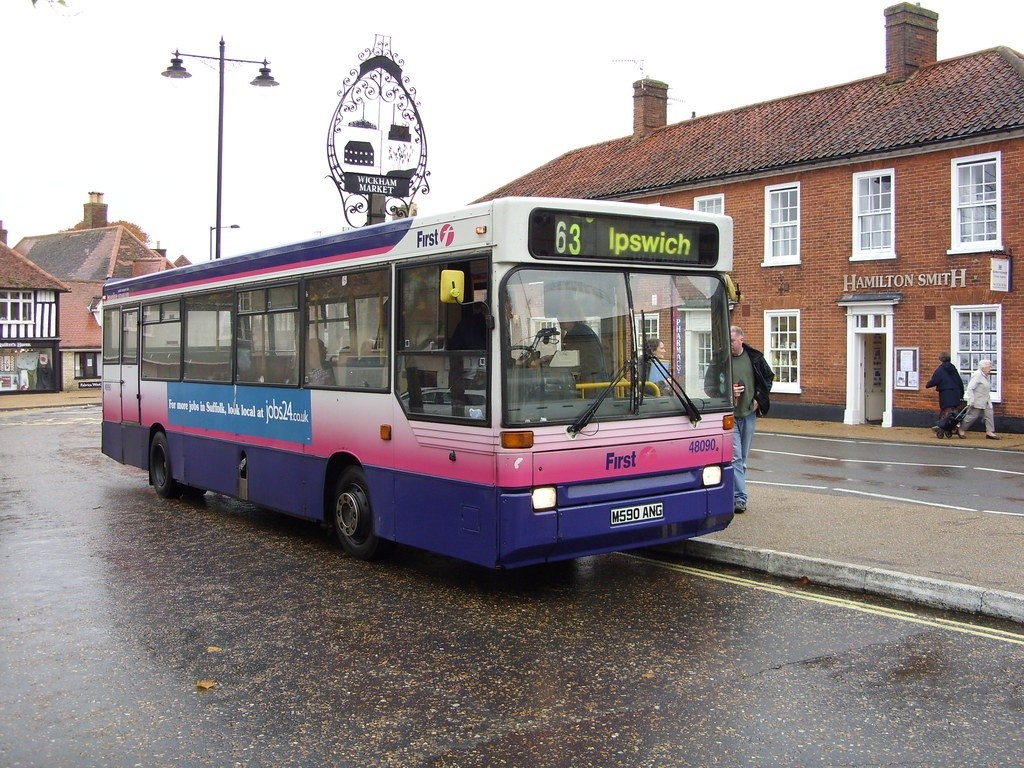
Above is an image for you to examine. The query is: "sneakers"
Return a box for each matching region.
[734,501,746,513]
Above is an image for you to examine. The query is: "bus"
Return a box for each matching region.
[96,192,736,574]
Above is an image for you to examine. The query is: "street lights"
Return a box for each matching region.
[209,224,241,260]
[158,35,281,260]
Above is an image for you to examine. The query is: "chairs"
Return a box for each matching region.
[118,338,377,387]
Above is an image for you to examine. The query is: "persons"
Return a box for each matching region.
[957,360,1000,440]
[446,295,531,404]
[638,339,675,398]
[704,326,774,513]
[308,337,375,386]
[557,299,612,399]
[926,352,964,430]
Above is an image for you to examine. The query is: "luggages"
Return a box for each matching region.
[936,405,974,439]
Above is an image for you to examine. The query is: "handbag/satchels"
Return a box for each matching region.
[958,398,967,414]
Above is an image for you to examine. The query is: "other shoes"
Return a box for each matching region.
[932,426,939,430]
[956,429,966,439]
[986,434,1000,440]
